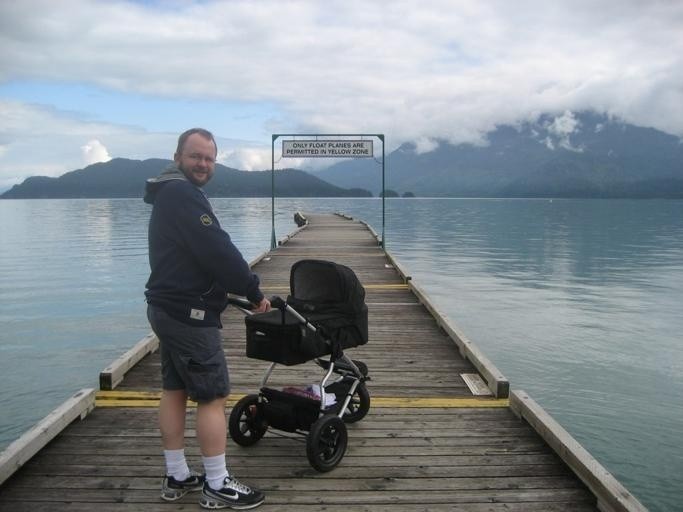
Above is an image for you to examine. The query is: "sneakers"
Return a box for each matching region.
[198,475,265,511]
[159,469,204,502]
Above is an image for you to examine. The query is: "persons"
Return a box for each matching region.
[144,128,272,509]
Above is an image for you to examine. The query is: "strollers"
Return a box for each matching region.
[226,257,372,473]
